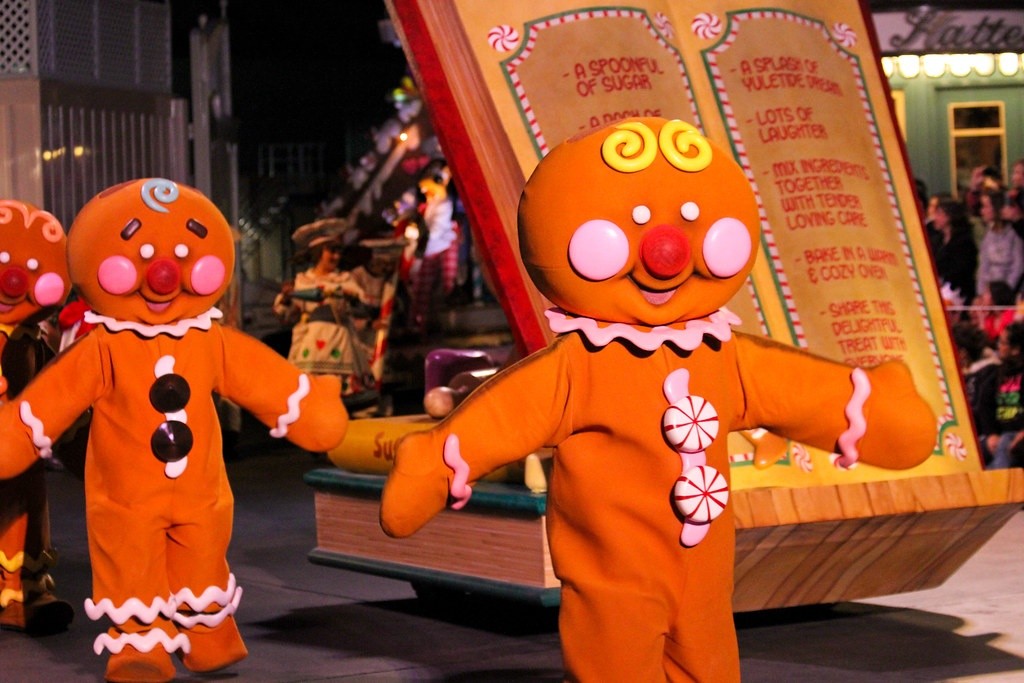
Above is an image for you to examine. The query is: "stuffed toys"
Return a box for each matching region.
[389,154,468,330]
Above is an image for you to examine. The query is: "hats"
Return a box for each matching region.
[359,240,410,260]
[291,220,349,249]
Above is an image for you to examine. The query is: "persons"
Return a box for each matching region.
[0,201,77,633]
[916,157,1024,467]
[0,178,349,683]
[273,218,410,421]
[379,115,938,683]
